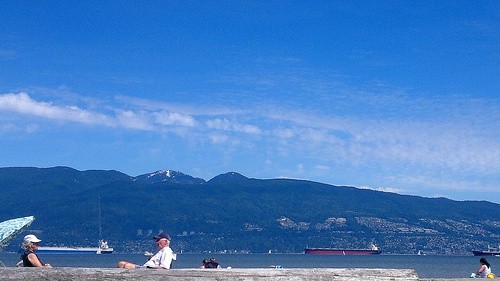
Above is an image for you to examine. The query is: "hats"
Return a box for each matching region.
[153,232,171,241]
[24,234,41,242]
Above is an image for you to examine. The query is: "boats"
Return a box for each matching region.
[304,246,383,256]
[35,247,114,255]
[472,245,500,256]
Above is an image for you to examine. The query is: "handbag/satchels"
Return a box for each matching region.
[487,273,495,279]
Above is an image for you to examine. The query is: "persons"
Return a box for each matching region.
[203,258,219,268]
[477,258,491,278]
[21,235,45,267]
[118,233,173,270]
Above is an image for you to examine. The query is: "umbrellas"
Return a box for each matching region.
[0,216,35,267]
